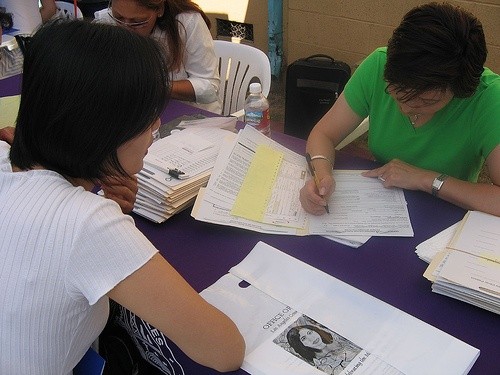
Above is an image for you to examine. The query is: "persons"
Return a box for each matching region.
[299,2,500,216]
[0,16,246,375]
[0,0,56,56]
[90,0,224,114]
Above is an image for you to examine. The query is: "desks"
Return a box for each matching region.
[0,60,500,375]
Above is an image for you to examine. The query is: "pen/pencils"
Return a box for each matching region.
[305,152,329,214]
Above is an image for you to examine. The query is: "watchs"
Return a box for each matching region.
[430,171,449,199]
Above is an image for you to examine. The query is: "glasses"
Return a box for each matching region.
[107,0,159,29]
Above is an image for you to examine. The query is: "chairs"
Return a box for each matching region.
[212,40,271,122]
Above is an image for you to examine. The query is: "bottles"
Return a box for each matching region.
[244,83,271,139]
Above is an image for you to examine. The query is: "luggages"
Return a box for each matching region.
[284,54,351,140]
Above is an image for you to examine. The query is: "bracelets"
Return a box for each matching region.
[311,155,334,170]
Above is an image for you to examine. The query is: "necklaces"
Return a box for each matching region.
[408,112,424,124]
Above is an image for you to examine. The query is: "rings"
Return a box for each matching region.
[377,174,385,182]
